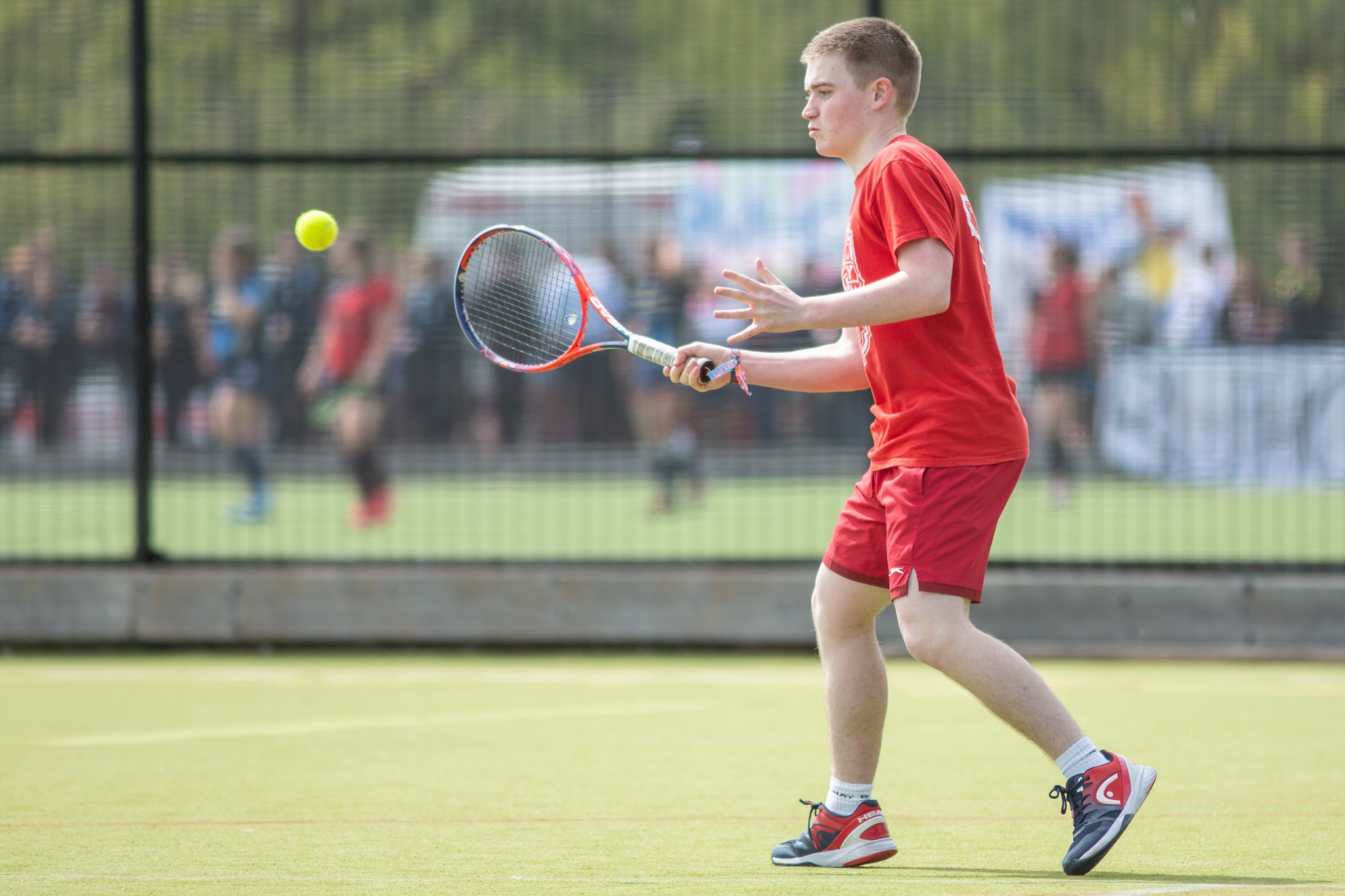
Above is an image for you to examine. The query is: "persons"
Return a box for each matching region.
[660,19,1157,876]
[0,216,877,524]
[1014,183,1345,501]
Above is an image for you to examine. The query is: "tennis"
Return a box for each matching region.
[295,208,338,252]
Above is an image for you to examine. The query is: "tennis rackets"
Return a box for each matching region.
[453,225,716,385]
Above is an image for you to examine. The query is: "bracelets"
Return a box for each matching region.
[706,348,752,397]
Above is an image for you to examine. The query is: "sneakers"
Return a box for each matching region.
[772,799,898,869]
[1049,750,1156,876]
